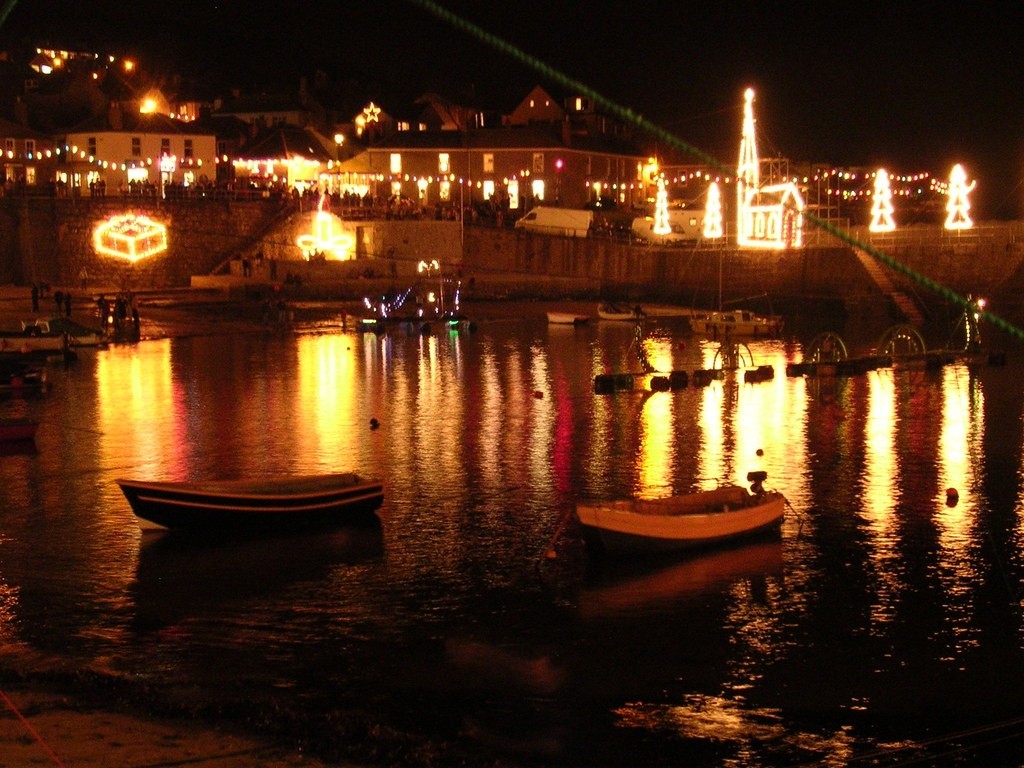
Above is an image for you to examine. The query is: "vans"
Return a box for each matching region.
[514,206,594,238]
[632,217,687,246]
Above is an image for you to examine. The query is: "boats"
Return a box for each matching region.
[691,309,785,338]
[572,470,785,541]
[114,473,386,526]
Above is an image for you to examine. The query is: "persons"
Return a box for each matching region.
[78,266,88,290]
[239,250,303,289]
[30,280,142,335]
[6,175,540,241]
